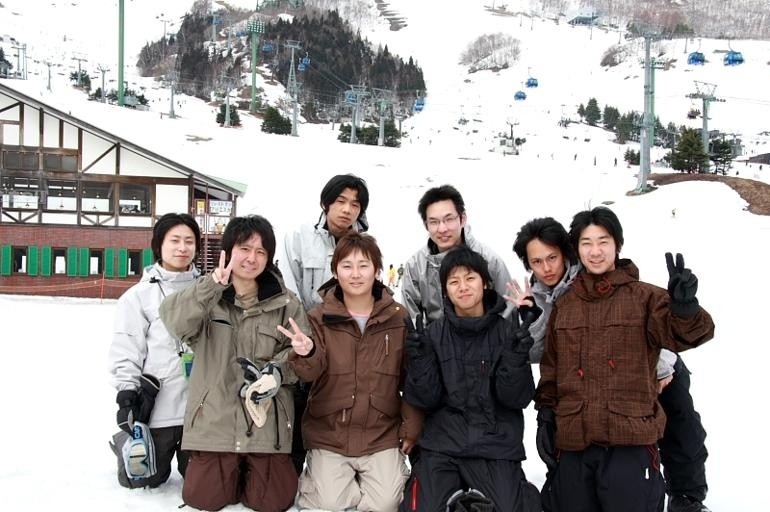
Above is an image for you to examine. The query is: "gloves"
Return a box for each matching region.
[239,363,282,402]
[535,408,560,471]
[237,356,263,399]
[137,373,160,423]
[114,391,140,435]
[503,308,534,366]
[664,251,700,316]
[403,313,432,360]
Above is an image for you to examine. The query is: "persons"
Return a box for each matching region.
[275,231,424,511]
[533,204,717,511]
[272,173,385,322]
[395,251,536,512]
[510,215,710,511]
[391,184,520,325]
[158,217,314,511]
[107,212,215,491]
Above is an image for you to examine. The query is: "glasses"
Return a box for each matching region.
[425,214,461,227]
[127,432,149,477]
